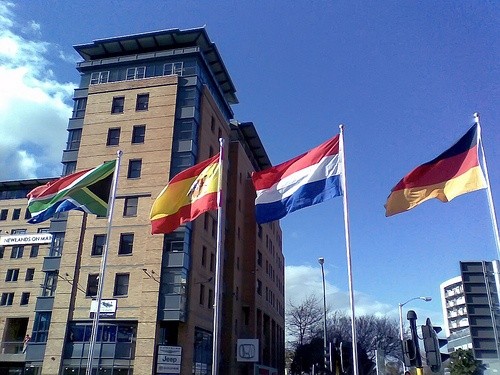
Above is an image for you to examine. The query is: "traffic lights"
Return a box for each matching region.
[309,363,321,375]
[337,341,353,373]
[322,342,336,372]
[421,318,450,373]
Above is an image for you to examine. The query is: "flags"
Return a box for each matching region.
[251,133,344,223]
[150,147,224,235]
[27,160,117,224]
[384,122,487,217]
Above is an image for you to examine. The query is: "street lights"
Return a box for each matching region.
[318,258,328,375]
[398,297,432,374]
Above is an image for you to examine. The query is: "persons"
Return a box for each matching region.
[22,334,30,354]
[405,371,411,375]
[2,338,8,354]
